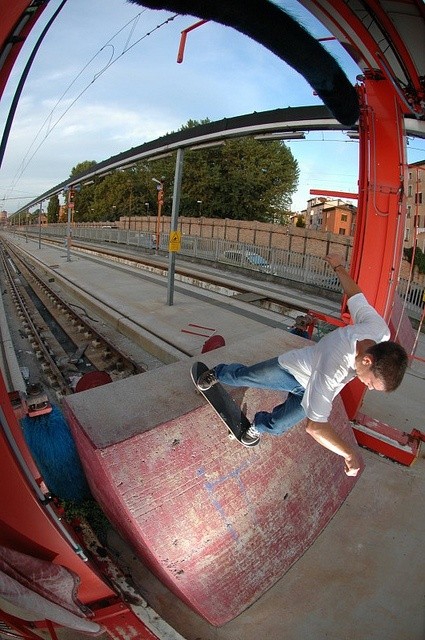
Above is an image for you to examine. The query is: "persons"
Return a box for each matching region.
[196,252,408,477]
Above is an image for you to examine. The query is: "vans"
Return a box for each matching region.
[221,249,273,274]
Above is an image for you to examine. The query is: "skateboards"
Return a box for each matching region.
[190,361,260,447]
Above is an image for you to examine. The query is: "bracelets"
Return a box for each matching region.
[333,264,344,272]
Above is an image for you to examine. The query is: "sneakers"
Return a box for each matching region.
[241,418,261,445]
[197,366,220,391]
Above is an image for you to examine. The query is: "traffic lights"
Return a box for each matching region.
[69,191,75,203]
